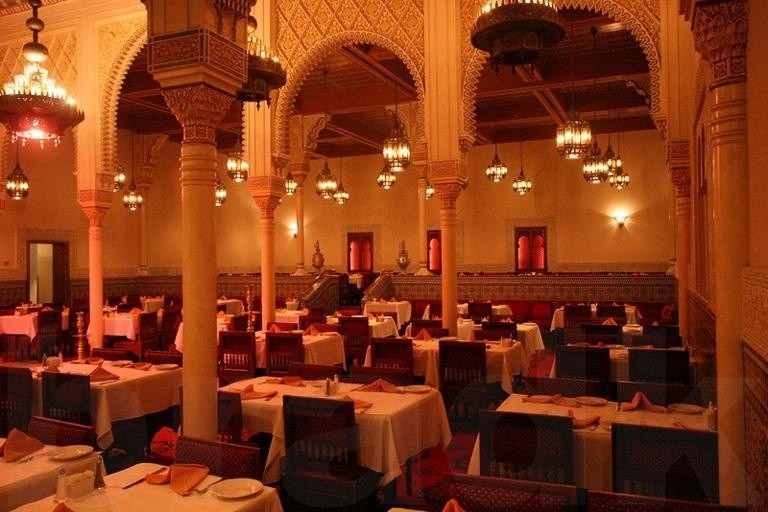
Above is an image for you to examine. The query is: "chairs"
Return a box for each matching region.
[1,294,717,512]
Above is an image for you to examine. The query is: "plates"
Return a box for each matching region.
[311,380,327,388]
[326,331,338,336]
[575,395,607,406]
[669,402,705,413]
[402,385,432,393]
[215,477,264,499]
[156,363,179,369]
[47,444,94,461]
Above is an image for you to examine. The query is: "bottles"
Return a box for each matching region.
[42,353,48,367]
[58,352,63,367]
[54,468,68,503]
[92,455,107,493]
[708,401,718,431]
[324,374,340,395]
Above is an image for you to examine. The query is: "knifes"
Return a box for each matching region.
[614,401,620,412]
[100,379,116,385]
[122,467,168,491]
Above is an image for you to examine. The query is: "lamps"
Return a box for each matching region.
[315,69,338,200]
[378,174,395,190]
[607,157,622,176]
[484,109,508,184]
[123,127,144,213]
[217,180,228,207]
[611,173,629,185]
[512,138,531,195]
[114,165,125,196]
[2,2,86,148]
[427,186,436,200]
[584,136,611,185]
[383,84,411,172]
[285,170,298,196]
[332,115,350,204]
[226,129,249,184]
[6,143,29,201]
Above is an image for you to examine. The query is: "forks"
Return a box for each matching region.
[194,478,229,493]
